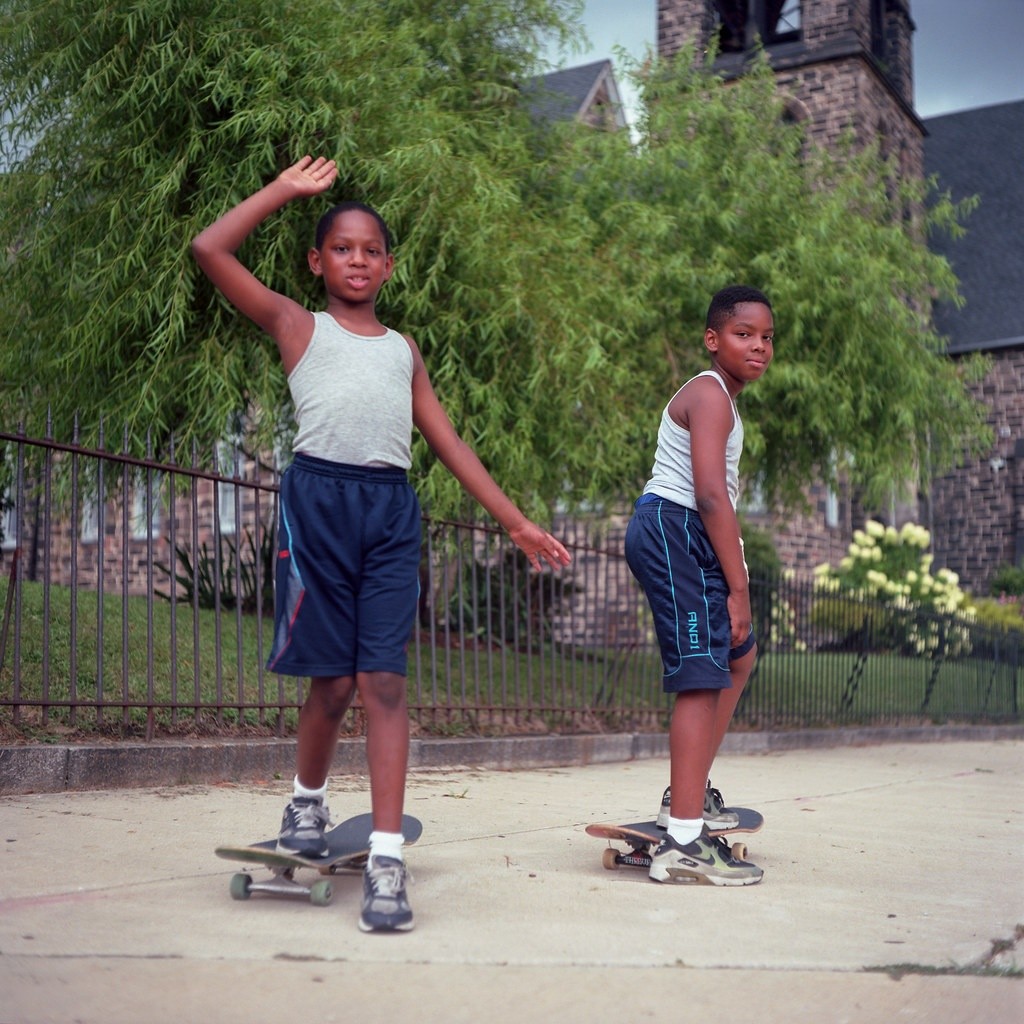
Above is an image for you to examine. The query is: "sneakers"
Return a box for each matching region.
[359,854,415,933]
[648,823,764,885]
[275,796,336,858]
[655,779,740,830]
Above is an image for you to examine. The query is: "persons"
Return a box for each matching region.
[186,151,573,936]
[620,286,776,888]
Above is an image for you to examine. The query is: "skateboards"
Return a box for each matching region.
[584,806,764,870]
[213,813,423,907]
